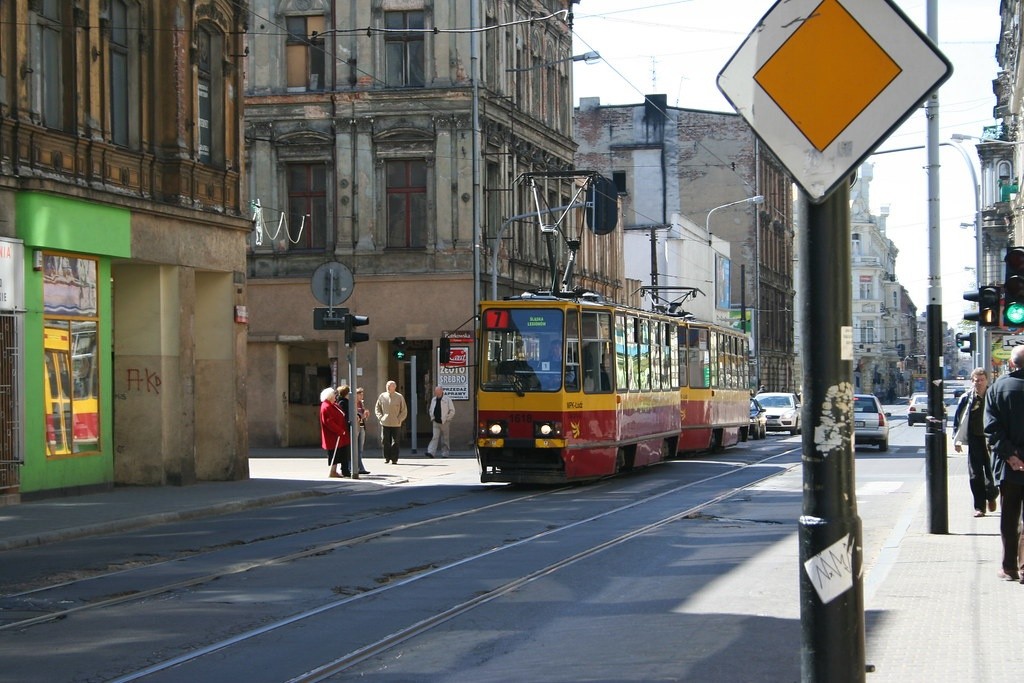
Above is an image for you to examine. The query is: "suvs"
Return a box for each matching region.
[853,393,892,451]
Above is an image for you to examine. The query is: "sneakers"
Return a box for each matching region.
[1001,569,1018,581]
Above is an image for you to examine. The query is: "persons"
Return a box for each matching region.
[425,386,456,458]
[537,340,576,389]
[983,344,1024,582]
[374,381,408,464]
[952,368,999,517]
[319,385,370,478]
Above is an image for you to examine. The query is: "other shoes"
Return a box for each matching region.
[359,470,370,474]
[973,510,985,517]
[392,461,398,464]
[987,499,996,513]
[329,472,343,478]
[385,457,390,463]
[424,452,434,458]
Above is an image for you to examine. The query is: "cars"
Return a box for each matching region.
[953,386,965,398]
[909,393,926,404]
[751,392,802,435]
[955,375,966,386]
[908,395,947,426]
[749,398,768,440]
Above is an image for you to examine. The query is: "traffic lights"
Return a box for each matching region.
[963,287,980,321]
[956,333,964,347]
[344,313,369,346]
[1002,246,1024,328]
[392,336,406,360]
[979,286,1000,326]
[960,332,977,356]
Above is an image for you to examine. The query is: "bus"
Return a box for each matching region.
[43,325,98,455]
[439,285,750,483]
[906,374,926,398]
